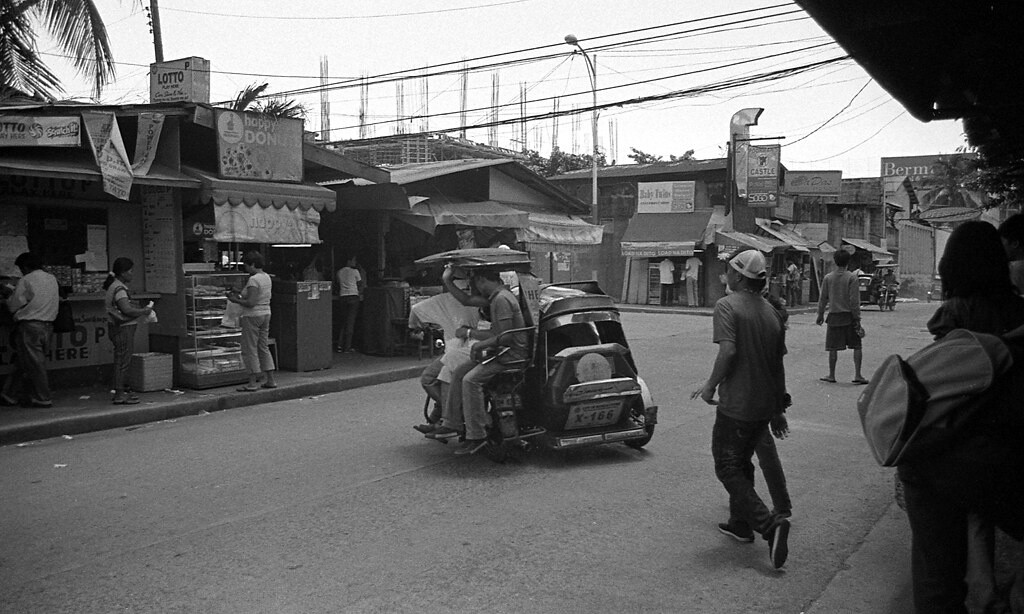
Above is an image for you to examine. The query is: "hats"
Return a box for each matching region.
[728,246,767,280]
[886,268,894,272]
[937,220,1011,282]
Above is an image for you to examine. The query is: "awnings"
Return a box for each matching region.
[842,237,896,261]
[0,147,604,244]
[716,224,819,253]
[620,208,712,258]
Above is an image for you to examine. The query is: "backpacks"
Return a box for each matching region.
[856,321,1023,467]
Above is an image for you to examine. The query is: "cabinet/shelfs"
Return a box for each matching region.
[176,271,262,389]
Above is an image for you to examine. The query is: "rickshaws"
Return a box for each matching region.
[852,261,903,312]
[413,243,660,464]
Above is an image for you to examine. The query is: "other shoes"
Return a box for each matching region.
[0,397,52,408]
[335,345,356,353]
[111,392,140,404]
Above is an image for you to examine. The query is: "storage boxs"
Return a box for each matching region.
[130,352,173,391]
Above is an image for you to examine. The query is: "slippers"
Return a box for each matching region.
[261,381,277,388]
[820,374,837,383]
[236,384,258,391]
[851,377,869,384]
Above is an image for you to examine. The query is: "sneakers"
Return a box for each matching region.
[718,523,755,544]
[425,426,461,440]
[453,437,487,455]
[768,519,791,570]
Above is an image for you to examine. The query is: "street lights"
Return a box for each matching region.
[559,34,606,229]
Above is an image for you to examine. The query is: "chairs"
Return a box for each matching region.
[391,288,437,361]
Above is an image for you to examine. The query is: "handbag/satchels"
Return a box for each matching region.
[108,285,142,323]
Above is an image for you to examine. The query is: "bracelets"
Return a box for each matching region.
[467,327,473,337]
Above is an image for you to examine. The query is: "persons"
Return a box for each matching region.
[409,264,527,455]
[852,265,897,303]
[335,248,368,353]
[897,211,1023,614]
[659,256,675,306]
[684,256,702,308]
[103,257,152,404]
[0,252,60,405]
[816,250,869,384]
[225,250,276,392]
[785,260,797,307]
[690,246,792,573]
